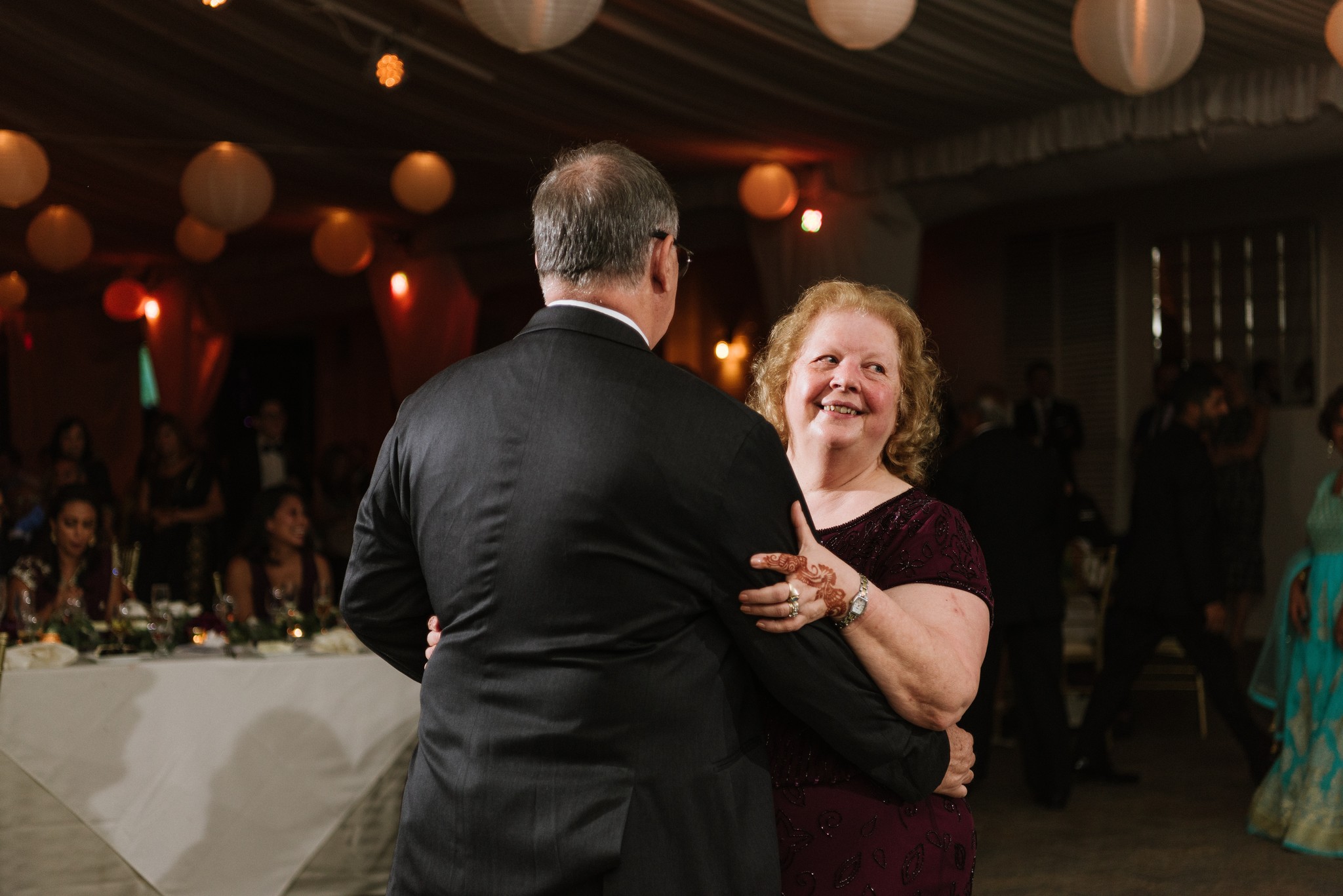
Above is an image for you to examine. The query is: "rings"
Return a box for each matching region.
[785,582,798,602]
[788,602,799,618]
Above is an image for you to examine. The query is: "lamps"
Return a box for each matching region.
[393,152,453,215]
[1071,0,1203,94]
[313,212,375,277]
[26,205,92,273]
[740,164,800,220]
[1323,1,1342,68]
[101,141,273,325]
[1,131,49,209]
[457,0,607,56]
[805,0,917,50]
[1,275,25,309]
[376,53,405,88]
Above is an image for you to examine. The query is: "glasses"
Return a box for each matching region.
[651,230,693,274]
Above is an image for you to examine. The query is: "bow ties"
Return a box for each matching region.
[258,443,282,453]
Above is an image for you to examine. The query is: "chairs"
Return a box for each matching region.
[1090,551,1214,742]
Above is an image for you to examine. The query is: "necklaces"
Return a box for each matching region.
[56,561,81,590]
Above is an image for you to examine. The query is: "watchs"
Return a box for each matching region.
[831,571,870,632]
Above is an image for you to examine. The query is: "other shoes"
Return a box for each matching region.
[1032,783,1066,808]
[1077,758,1136,783]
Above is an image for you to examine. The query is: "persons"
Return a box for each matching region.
[1016,359,1087,464]
[215,394,304,569]
[139,416,224,596]
[1130,364,1180,509]
[47,453,82,495]
[1237,381,1343,859]
[44,412,112,531]
[932,385,1075,808]
[1200,353,1268,592]
[222,483,330,634]
[729,275,990,896]
[1,482,120,641]
[1080,365,1284,784]
[340,142,978,896]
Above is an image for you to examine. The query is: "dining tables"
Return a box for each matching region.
[1,623,431,896]
